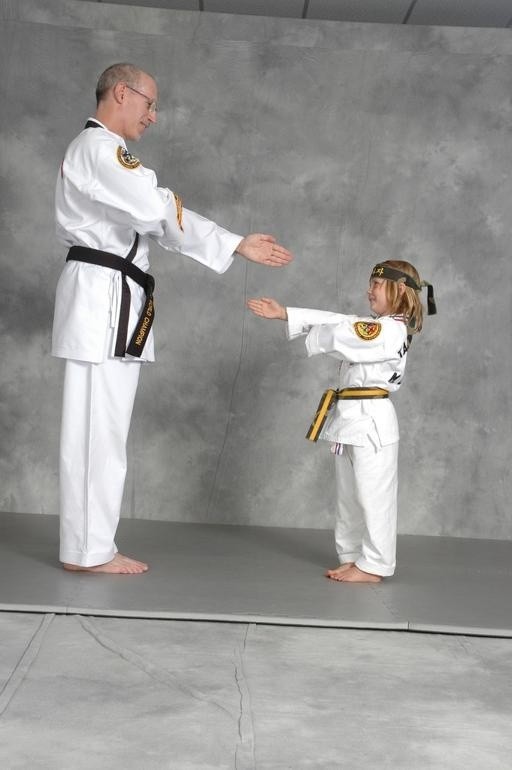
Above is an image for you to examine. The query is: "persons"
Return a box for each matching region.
[48,61,295,574]
[246,260,438,583]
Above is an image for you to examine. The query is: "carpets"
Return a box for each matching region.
[1,508,512,641]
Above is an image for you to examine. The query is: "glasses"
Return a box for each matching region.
[124,85,156,114]
